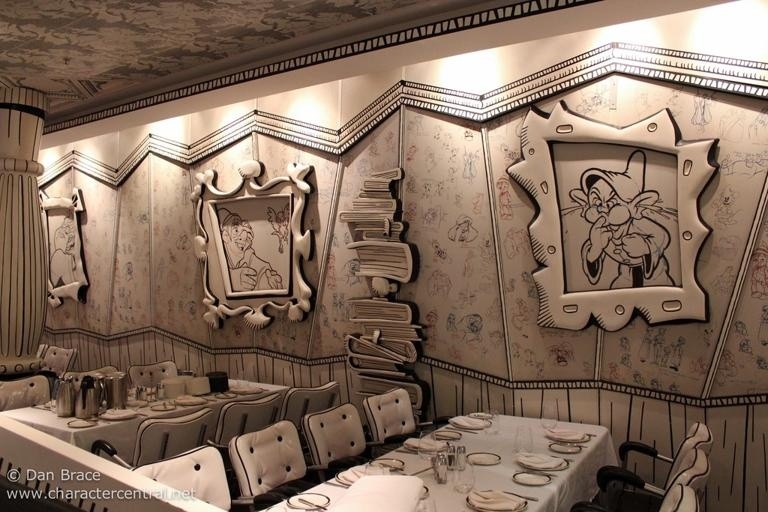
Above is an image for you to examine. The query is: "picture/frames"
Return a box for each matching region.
[38,187,91,309]
[189,160,315,330]
[504,98,722,333]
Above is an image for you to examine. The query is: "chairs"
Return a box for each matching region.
[361,387,455,453]
[228,419,352,511]
[214,392,282,445]
[596,421,714,492]
[40,344,80,380]
[576,448,711,511]
[36,343,49,360]
[128,444,283,511]
[278,381,340,440]
[63,365,120,385]
[0,375,52,412]
[569,483,700,512]
[300,402,419,483]
[91,407,214,468]
[128,359,178,387]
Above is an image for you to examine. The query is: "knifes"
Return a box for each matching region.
[502,490,539,502]
[444,427,478,434]
[297,498,327,511]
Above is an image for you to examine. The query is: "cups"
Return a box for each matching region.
[414,494,437,512]
[540,403,559,430]
[113,399,122,414]
[451,464,476,494]
[417,429,439,459]
[482,408,500,434]
[364,464,385,475]
[513,424,535,455]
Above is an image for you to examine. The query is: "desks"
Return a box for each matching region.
[254,412,619,511]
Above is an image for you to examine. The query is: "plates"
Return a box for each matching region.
[334,469,365,486]
[446,417,493,430]
[402,441,439,453]
[98,409,139,421]
[514,455,570,472]
[229,387,264,395]
[368,457,406,471]
[549,442,583,454]
[421,485,429,501]
[464,489,530,512]
[430,430,463,441]
[466,451,503,466]
[66,418,98,429]
[286,492,331,511]
[149,403,176,411]
[511,470,553,487]
[543,433,592,443]
[171,399,209,407]
[469,411,493,419]
[214,391,238,400]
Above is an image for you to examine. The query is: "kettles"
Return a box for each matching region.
[75,375,98,419]
[52,376,76,417]
[99,374,127,410]
